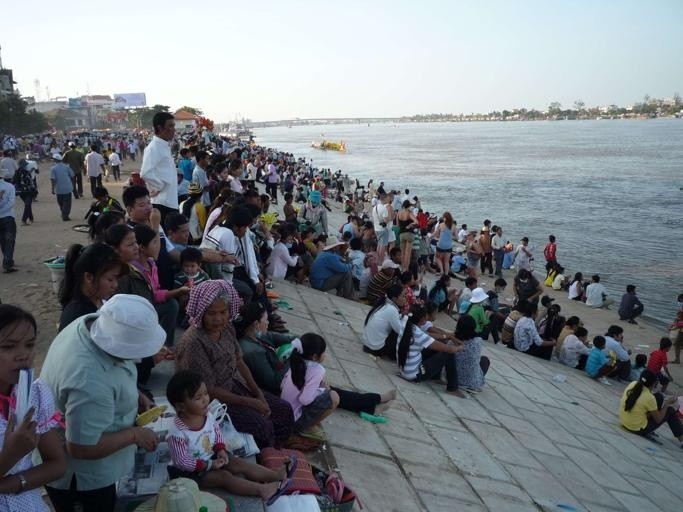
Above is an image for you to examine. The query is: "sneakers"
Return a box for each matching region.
[597,376,612,386]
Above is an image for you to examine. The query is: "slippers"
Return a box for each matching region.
[265,476,294,507]
[281,455,299,479]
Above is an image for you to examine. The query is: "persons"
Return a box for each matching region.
[0,113,683,512]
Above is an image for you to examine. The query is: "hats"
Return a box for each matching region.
[68,141,76,147]
[468,287,490,303]
[17,158,29,169]
[187,180,204,195]
[322,236,345,251]
[541,295,555,306]
[87,293,168,359]
[382,259,400,270]
[132,476,230,511]
[49,153,63,161]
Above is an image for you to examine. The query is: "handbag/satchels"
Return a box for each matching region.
[314,472,355,512]
